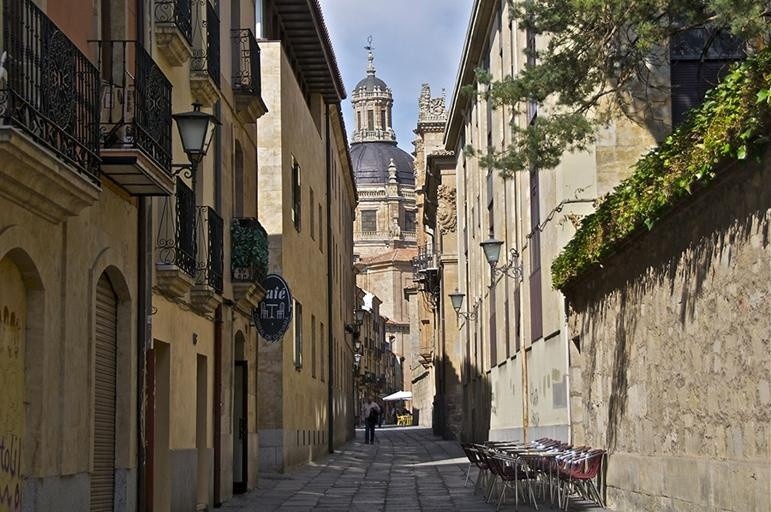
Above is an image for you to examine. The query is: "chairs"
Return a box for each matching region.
[459,437,607,512]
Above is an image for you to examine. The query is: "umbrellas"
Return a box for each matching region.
[382,390,412,409]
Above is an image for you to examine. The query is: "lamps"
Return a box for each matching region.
[170,100,222,179]
[352,301,367,352]
[480,227,524,280]
[448,287,478,322]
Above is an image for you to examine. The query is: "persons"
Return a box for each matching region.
[391,408,411,425]
[361,395,381,445]
